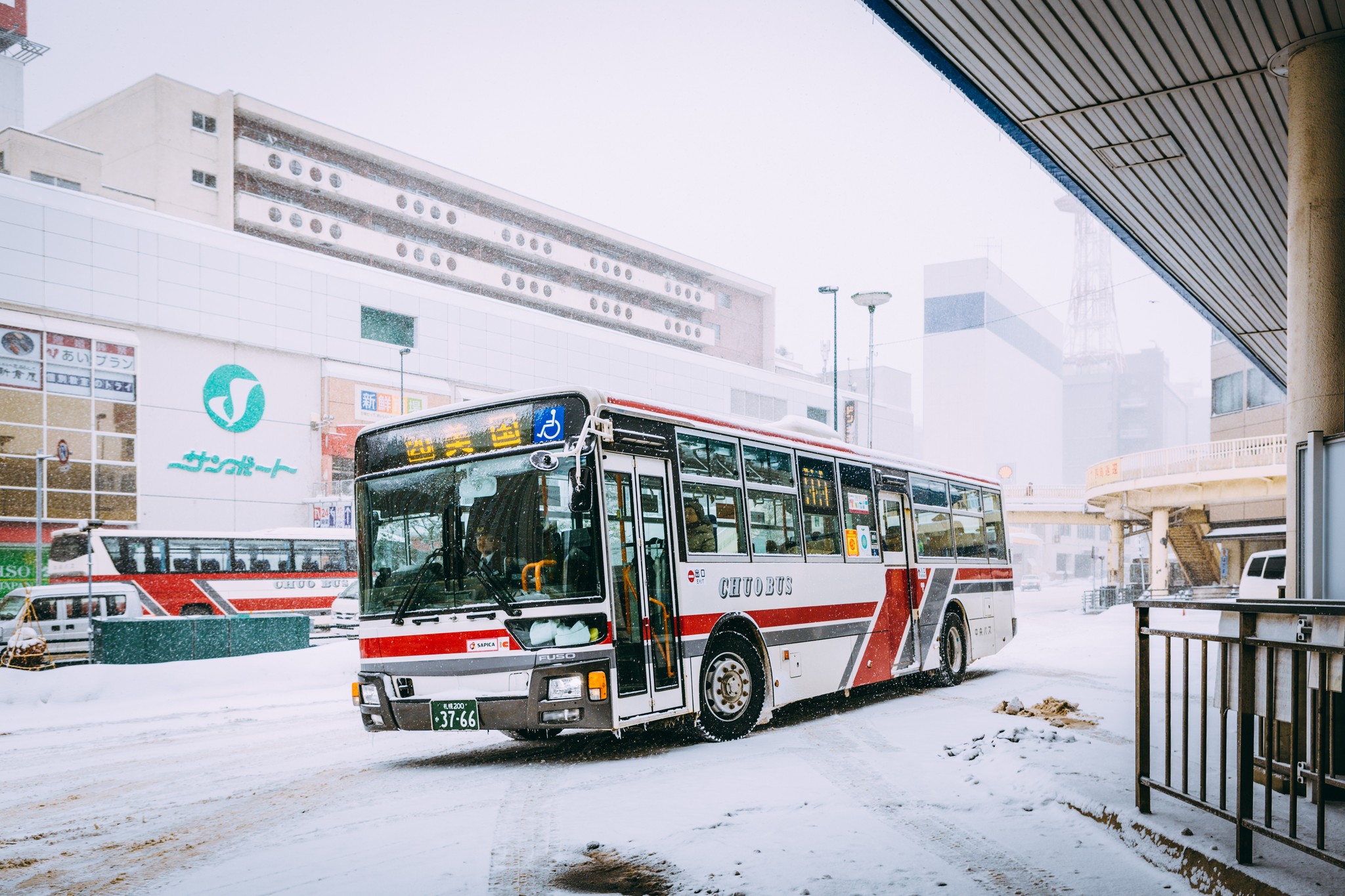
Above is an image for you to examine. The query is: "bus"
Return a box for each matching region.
[351,385,1018,743]
[46,527,357,629]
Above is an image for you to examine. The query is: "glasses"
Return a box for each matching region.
[684,513,696,516]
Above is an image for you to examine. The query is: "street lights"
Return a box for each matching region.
[818,285,841,433]
[78,519,106,664]
[400,348,411,415]
[849,291,893,450]
[1099,556,1105,606]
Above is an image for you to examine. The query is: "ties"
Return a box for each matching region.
[481,558,487,569]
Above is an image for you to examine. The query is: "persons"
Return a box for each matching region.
[681,500,717,554]
[462,524,523,594]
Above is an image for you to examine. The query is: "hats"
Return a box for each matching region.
[475,524,492,536]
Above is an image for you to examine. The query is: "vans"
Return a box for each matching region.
[1238,548,1286,599]
[331,578,378,640]
[0,582,143,646]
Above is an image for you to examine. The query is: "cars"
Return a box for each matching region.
[1020,575,1042,591]
